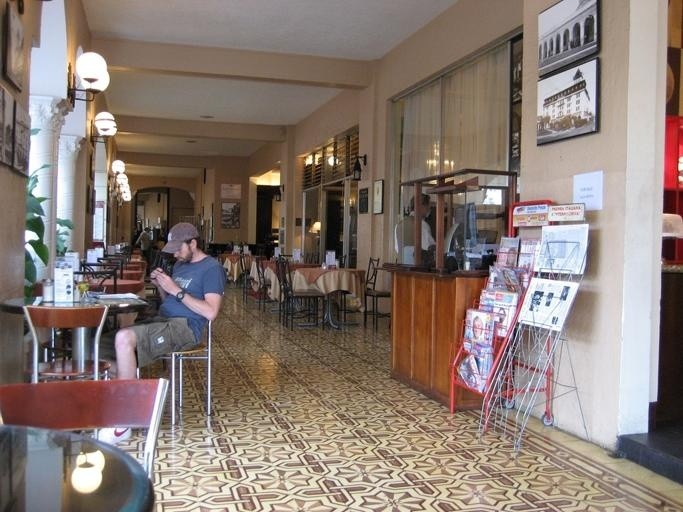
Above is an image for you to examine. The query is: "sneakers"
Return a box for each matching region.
[98,427,131,444]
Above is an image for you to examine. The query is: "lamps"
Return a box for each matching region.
[274,184,284,201]
[353,154,367,181]
[68,52,132,207]
[661,214,683,261]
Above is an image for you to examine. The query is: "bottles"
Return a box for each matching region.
[41,277,54,303]
[73,285,82,303]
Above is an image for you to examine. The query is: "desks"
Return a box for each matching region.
[0,423,155,512]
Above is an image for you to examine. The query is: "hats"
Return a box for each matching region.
[162,222,199,254]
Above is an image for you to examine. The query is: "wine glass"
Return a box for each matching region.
[452,244,466,271]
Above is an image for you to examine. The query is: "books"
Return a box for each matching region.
[455,223,590,393]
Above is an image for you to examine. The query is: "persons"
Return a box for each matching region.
[92,222,226,445]
[135,227,152,277]
[393,193,436,265]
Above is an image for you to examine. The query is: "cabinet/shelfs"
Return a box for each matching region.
[648,271,683,430]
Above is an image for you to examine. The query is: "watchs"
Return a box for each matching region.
[175,289,186,303]
